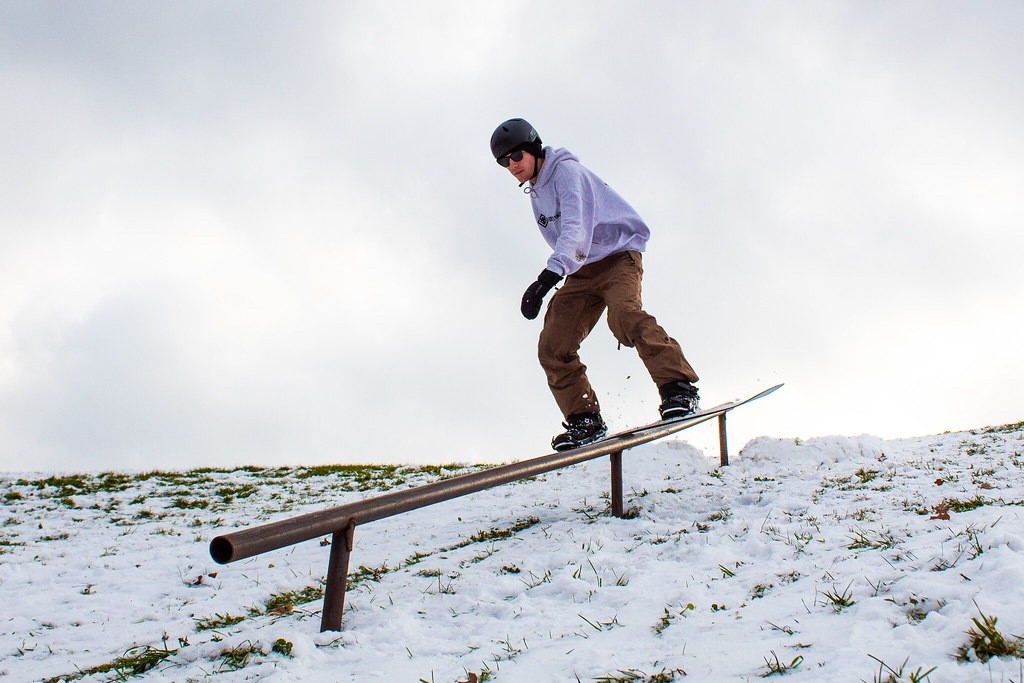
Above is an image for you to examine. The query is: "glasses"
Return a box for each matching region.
[497,147,523,167]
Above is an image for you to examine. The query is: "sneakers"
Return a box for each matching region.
[658,380,700,420]
[551,410,608,452]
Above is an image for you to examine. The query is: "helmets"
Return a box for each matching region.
[491,118,542,159]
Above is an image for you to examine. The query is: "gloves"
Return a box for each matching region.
[521,268,564,320]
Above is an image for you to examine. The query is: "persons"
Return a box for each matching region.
[490,117,700,452]
